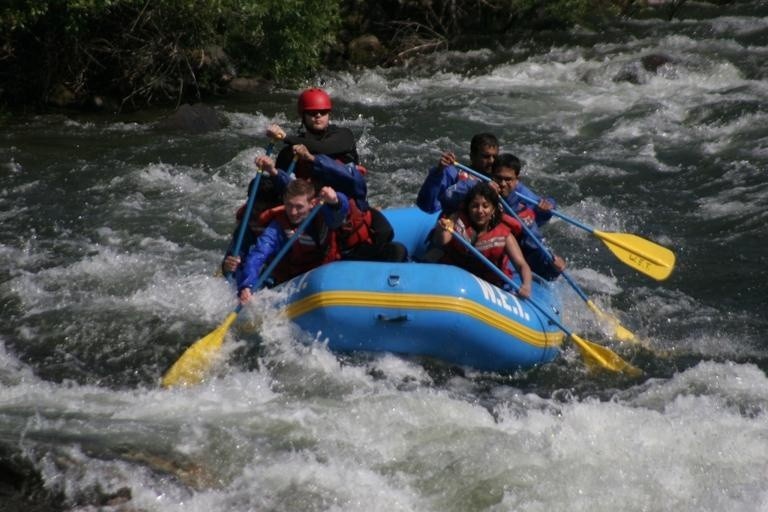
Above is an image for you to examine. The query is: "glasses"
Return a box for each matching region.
[305,111,329,116]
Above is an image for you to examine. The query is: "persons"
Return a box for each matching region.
[248,89,358,213]
[435,183,531,299]
[226,145,403,273]
[438,153,564,282]
[417,133,555,228]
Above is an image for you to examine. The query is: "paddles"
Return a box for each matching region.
[446,229,642,373]
[453,161,675,280]
[162,198,325,384]
[497,191,637,340]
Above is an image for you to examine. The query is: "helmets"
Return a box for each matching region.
[299,89,332,111]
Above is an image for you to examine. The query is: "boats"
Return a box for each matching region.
[233,200,564,373]
